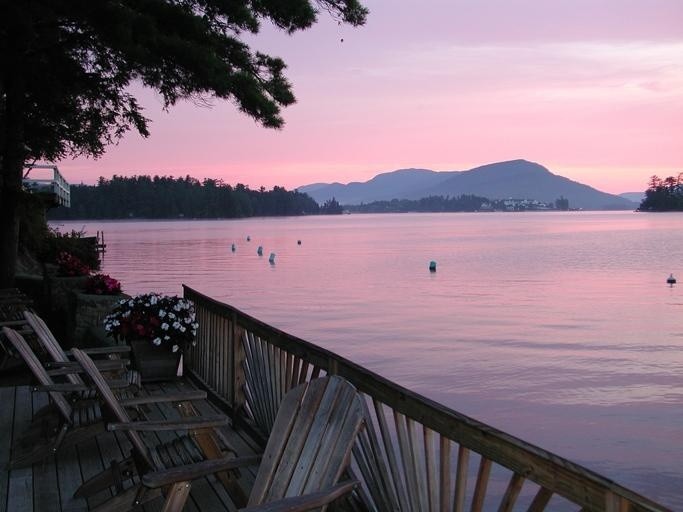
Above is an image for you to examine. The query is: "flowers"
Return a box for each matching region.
[84,274,123,295]
[53,252,91,276]
[102,292,200,354]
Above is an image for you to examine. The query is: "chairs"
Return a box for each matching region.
[71,346,266,511]
[1,309,151,453]
[140,375,365,511]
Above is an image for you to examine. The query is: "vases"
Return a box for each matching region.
[70,290,132,349]
[48,273,96,317]
